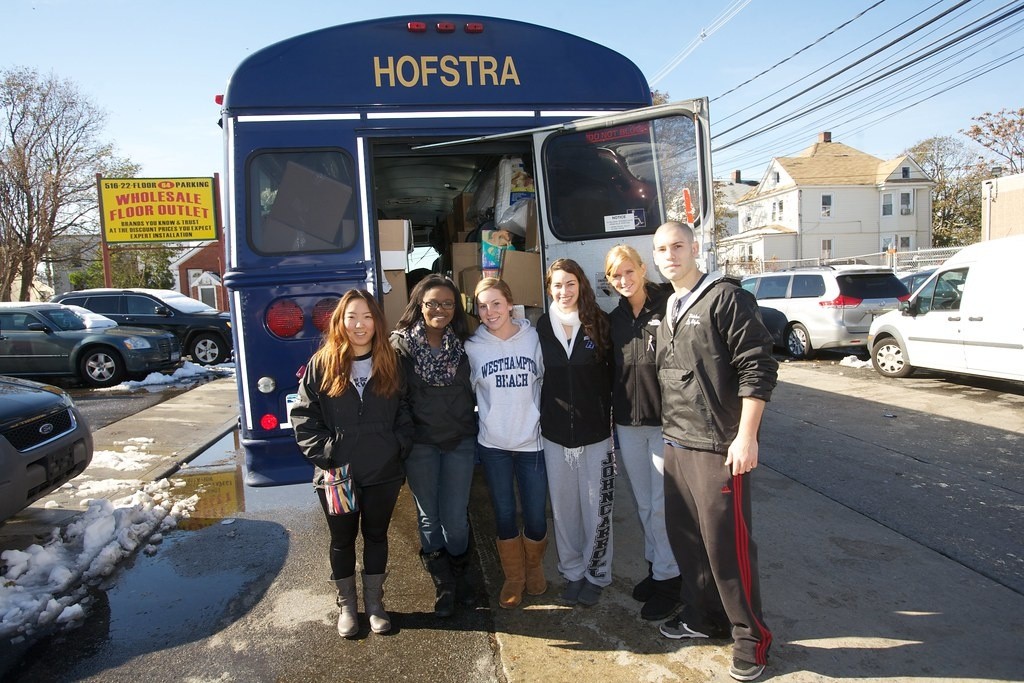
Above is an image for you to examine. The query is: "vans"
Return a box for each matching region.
[742,266,911,359]
[867,234,1024,386]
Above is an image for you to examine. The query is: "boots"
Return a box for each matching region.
[325,569,360,637]
[360,568,392,634]
[521,525,549,596]
[495,529,527,609]
[443,540,482,608]
[418,545,457,619]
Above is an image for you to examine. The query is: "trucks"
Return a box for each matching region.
[217,9,714,488]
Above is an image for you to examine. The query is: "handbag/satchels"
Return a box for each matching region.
[323,462,360,517]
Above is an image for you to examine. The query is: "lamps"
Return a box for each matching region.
[992,167,1002,178]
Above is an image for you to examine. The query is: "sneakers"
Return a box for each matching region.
[729,653,766,681]
[658,614,732,640]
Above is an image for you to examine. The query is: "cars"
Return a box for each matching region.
[900,268,965,308]
[0,302,183,387]
[0,376,93,524]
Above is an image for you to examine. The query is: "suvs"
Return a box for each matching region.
[49,289,232,366]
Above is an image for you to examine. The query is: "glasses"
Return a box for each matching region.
[422,300,456,310]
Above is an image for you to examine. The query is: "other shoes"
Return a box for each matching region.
[560,576,586,605]
[632,560,657,602]
[640,574,684,621]
[578,578,604,605]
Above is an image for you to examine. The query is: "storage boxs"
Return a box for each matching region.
[256,154,544,344]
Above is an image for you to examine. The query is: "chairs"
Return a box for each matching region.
[24,313,53,331]
[801,280,818,296]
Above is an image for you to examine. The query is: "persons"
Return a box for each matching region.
[652,220,779,681]
[536,258,615,607]
[388,274,477,612]
[606,246,685,621]
[290,289,418,638]
[463,277,547,609]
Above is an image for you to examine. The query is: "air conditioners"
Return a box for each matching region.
[747,254,752,261]
[901,209,912,215]
